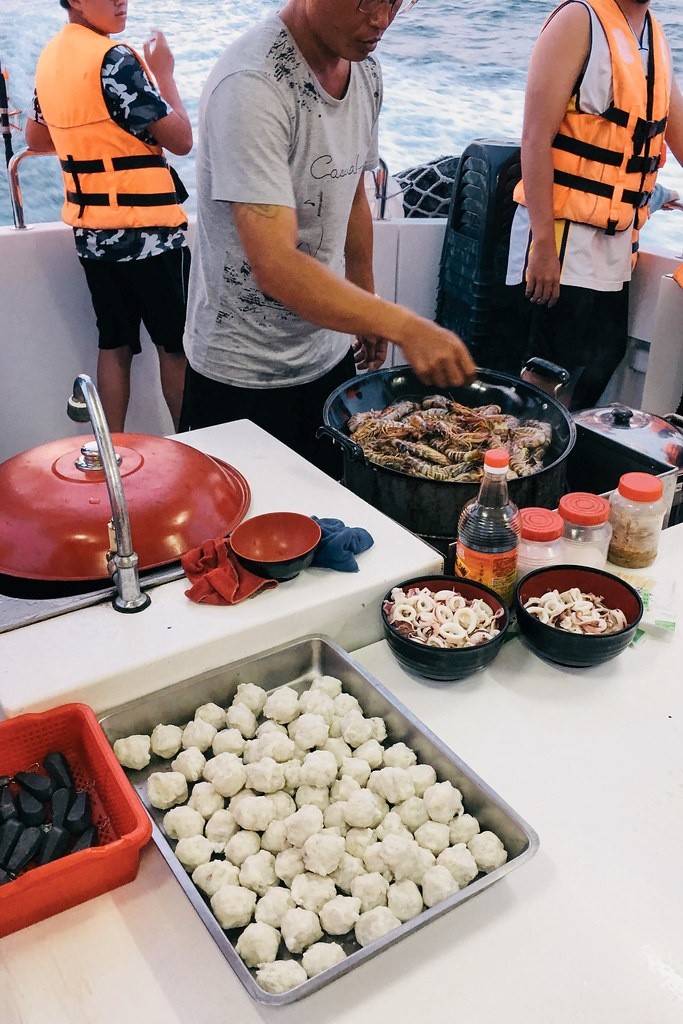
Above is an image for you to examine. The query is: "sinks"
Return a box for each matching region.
[0,560,186,633]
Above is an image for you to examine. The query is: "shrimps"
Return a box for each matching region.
[346,395,552,483]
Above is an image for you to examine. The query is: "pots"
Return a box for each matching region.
[317,357,577,544]
[572,405,683,477]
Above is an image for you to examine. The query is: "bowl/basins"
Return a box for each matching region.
[516,565,643,667]
[230,512,321,582]
[380,575,509,681]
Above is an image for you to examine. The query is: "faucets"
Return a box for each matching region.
[67,374,152,614]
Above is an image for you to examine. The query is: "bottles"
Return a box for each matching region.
[515,508,565,580]
[558,493,613,571]
[455,449,521,608]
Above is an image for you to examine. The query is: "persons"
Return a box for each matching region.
[179,0,479,464]
[24,0,193,435]
[506,1,683,408]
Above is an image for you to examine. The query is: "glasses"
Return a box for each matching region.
[358,0,419,16]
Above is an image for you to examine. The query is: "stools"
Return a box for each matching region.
[433,135,517,372]
